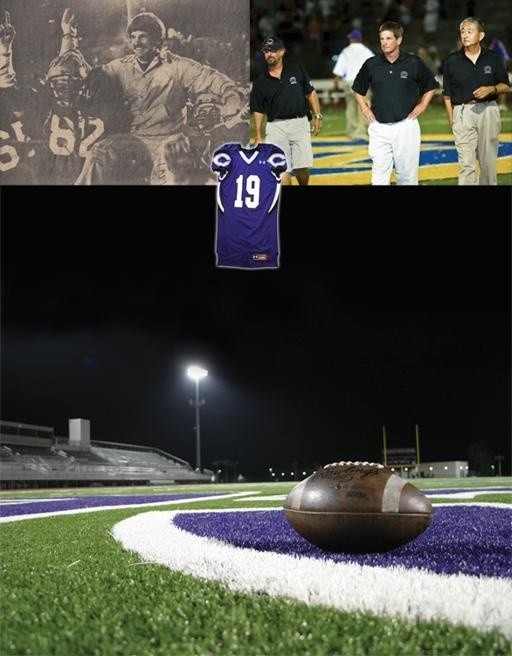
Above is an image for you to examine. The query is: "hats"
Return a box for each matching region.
[348,30,363,41]
[127,12,165,41]
[261,37,285,51]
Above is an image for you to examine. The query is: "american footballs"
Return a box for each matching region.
[283,464,433,553]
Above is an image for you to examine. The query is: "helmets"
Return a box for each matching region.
[46,49,87,80]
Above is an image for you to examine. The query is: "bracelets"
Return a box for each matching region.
[493,86,498,95]
[314,113,323,120]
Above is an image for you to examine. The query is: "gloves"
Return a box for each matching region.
[60,8,79,36]
[0,11,16,45]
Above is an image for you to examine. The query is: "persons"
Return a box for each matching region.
[332,30,376,142]
[250,35,322,186]
[442,17,512,186]
[351,21,440,186]
